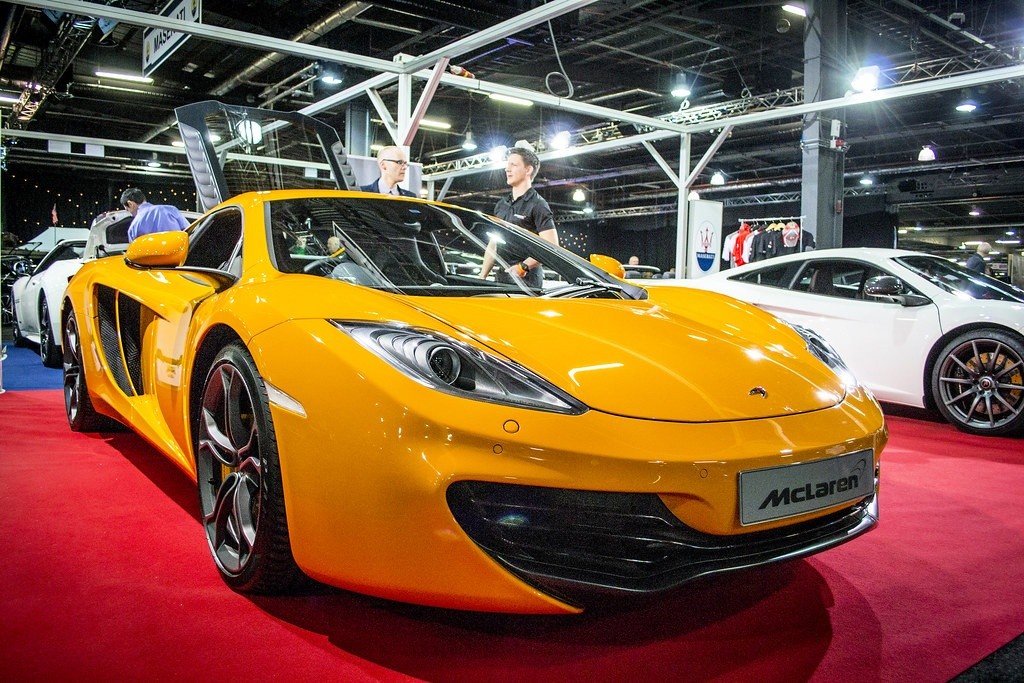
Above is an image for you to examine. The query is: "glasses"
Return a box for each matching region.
[386,159,407,167]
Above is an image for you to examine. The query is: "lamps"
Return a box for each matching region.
[44,0,980,216]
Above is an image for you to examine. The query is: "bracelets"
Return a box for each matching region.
[517,262,529,275]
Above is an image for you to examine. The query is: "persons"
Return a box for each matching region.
[983,267,996,298]
[960,242,992,299]
[120,188,190,244]
[360,146,417,198]
[624,256,642,279]
[480,148,559,288]
[325,236,340,256]
[289,234,316,255]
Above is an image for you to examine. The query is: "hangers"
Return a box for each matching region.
[736,215,801,234]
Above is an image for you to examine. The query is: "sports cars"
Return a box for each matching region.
[623,246,1024,433]
[445,251,571,288]
[61,100,889,616]
[10,208,206,368]
